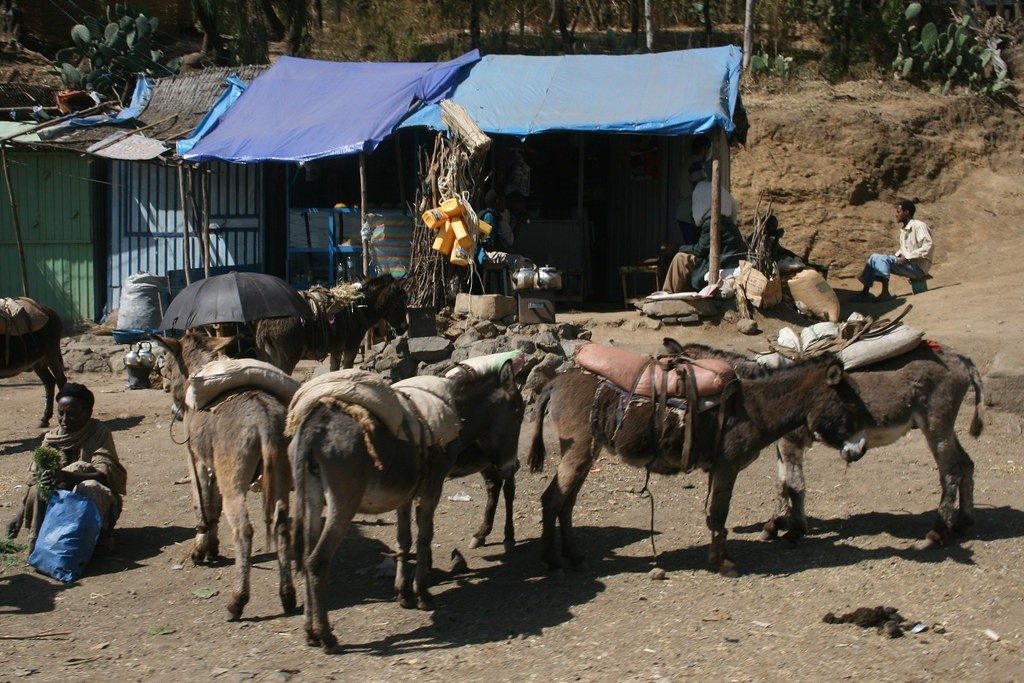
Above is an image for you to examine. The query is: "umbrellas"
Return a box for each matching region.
[157,270,314,352]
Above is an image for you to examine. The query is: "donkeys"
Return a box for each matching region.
[287,338,985,656]
[149,331,297,621]
[0,297,67,428]
[254,272,411,376]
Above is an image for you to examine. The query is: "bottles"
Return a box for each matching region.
[294,264,314,284]
[336,253,357,286]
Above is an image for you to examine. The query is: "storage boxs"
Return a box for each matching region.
[739,259,783,307]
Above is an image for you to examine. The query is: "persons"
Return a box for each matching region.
[474,191,518,298]
[748,214,827,280]
[848,201,933,304]
[661,161,745,292]
[5,382,126,555]
[676,134,714,246]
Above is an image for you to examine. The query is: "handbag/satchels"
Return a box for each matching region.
[26,488,101,583]
[739,258,783,309]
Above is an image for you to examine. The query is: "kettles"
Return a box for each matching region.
[533,264,563,290]
[125,342,143,366]
[138,341,156,367]
[508,258,538,290]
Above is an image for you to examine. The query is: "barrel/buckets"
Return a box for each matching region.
[409,306,436,337]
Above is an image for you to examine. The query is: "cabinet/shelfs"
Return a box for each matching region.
[511,219,584,308]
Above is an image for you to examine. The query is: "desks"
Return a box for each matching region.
[619,265,659,309]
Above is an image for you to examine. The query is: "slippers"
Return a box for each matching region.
[847,294,874,302]
[94,537,114,555]
[874,294,896,303]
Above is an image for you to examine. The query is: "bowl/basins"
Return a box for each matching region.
[112,329,146,343]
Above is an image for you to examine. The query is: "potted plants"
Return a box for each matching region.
[52,2,184,115]
